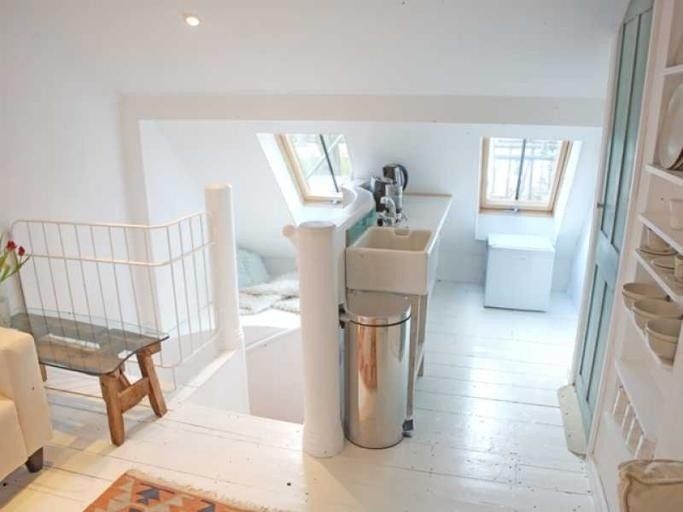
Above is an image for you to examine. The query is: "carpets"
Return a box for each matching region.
[83,469,291,512]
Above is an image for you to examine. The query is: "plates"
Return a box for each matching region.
[639,247,679,257]
[666,274,683,292]
[658,82,683,170]
[651,257,674,272]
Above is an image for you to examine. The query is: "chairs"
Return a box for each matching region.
[0,325,53,481]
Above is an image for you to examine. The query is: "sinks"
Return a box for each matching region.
[344,225,439,295]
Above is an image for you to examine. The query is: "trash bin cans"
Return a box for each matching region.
[339,294,415,450]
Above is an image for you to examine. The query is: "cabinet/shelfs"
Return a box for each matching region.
[557,0,683,512]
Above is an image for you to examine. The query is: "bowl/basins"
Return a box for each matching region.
[643,317,683,365]
[620,282,670,313]
[632,297,682,334]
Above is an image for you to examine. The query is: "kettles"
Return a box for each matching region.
[382,162,408,220]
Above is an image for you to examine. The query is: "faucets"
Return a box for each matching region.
[378,197,399,226]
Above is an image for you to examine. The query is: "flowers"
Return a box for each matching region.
[0,232,31,283]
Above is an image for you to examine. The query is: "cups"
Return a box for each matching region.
[370,175,392,211]
[668,198,683,231]
[674,254,683,283]
[647,229,672,252]
[612,386,657,462]
